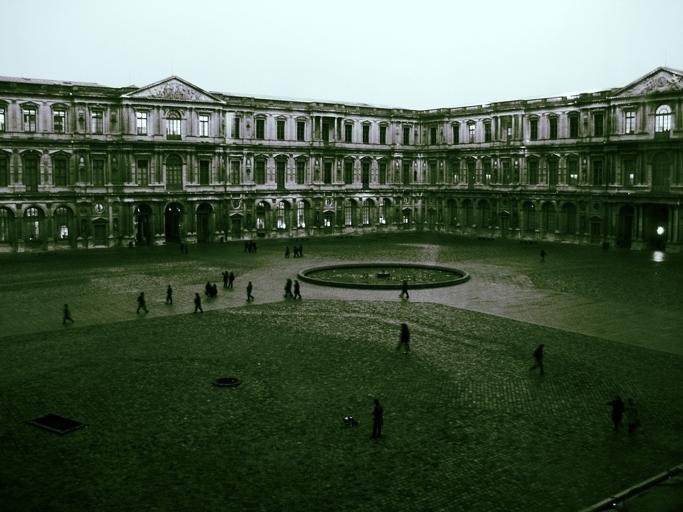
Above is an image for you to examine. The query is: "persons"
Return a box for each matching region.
[62,304,74,324]
[165,284,172,304]
[528,343,544,375]
[540,250,546,261]
[181,240,303,314]
[371,399,384,438]
[607,397,623,431]
[624,397,640,432]
[396,323,409,354]
[137,291,148,314]
[399,279,409,298]
